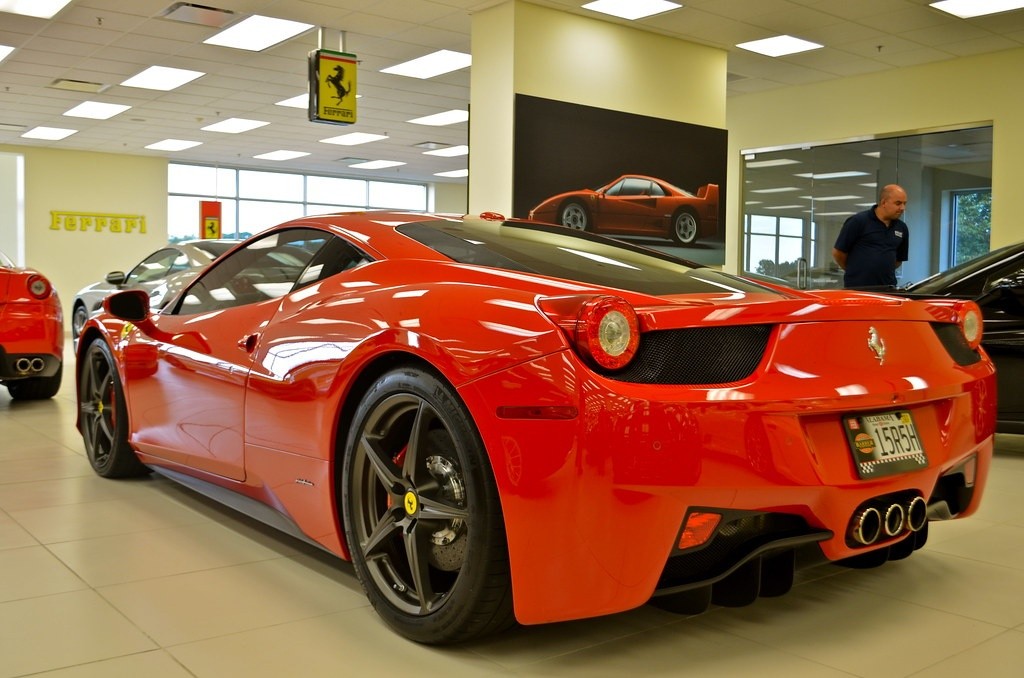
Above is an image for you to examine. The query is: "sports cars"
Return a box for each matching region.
[75,209,1003,650]
[527,174,720,248]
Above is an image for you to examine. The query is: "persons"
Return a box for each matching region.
[832,184,909,291]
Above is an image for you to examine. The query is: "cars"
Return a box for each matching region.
[892,240,1024,438]
[0,250,65,401]
[69,237,315,359]
